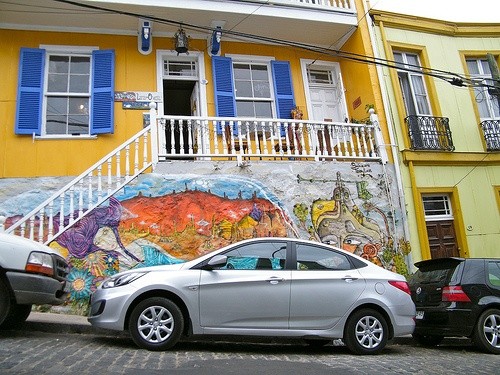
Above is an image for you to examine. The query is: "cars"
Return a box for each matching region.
[408,256,500,354]
[86,236,416,351]
[0,233,69,330]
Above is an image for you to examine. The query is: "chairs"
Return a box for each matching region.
[225,125,250,160]
[274,126,300,160]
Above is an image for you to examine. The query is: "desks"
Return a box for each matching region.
[244,131,271,161]
